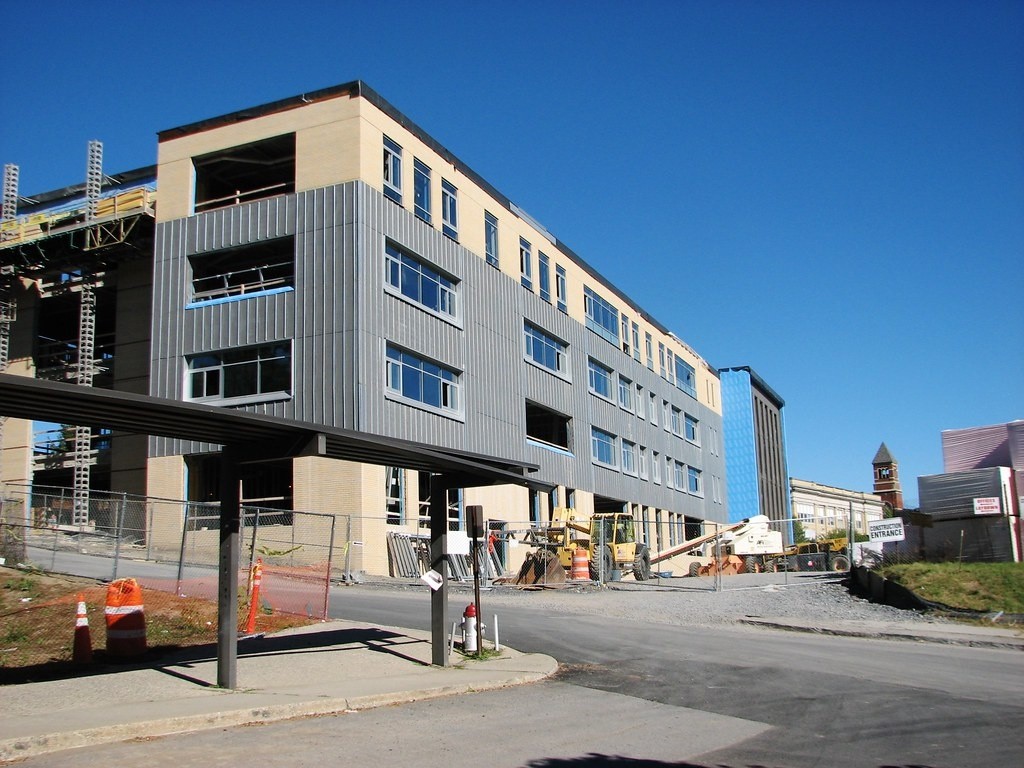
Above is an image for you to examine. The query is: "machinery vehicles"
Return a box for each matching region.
[541,504,651,585]
[778,534,852,575]
[622,514,784,575]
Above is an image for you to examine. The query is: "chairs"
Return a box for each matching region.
[613,529,626,543]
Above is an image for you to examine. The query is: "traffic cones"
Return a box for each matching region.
[74,596,94,662]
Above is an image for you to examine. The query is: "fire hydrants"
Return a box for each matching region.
[460,602,486,654]
